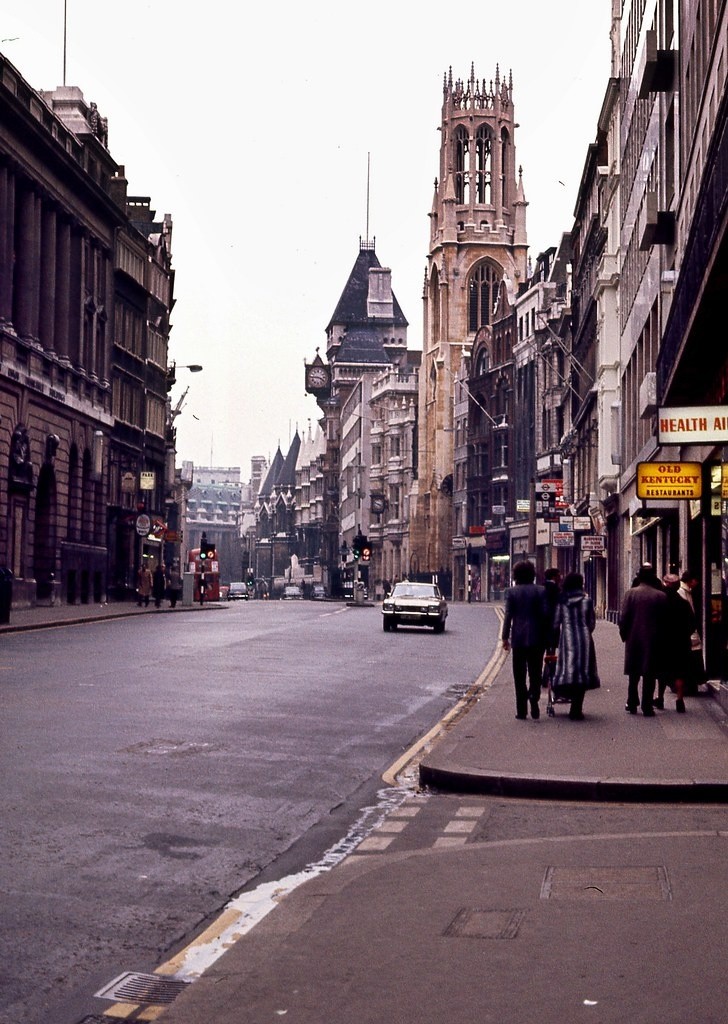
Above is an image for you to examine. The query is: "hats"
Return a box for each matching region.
[561,573,583,589]
[680,568,694,583]
[665,574,679,583]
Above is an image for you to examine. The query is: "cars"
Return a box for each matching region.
[281,586,302,601]
[382,582,448,635]
[311,585,326,602]
[226,582,249,601]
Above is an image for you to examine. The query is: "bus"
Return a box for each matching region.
[189,548,220,602]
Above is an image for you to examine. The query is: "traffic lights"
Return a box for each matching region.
[207,543,215,559]
[362,543,373,562]
[352,536,363,560]
[200,538,208,560]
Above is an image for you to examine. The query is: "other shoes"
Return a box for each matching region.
[530,696,540,720]
[676,698,685,713]
[625,703,638,715]
[570,713,585,721]
[643,710,655,718]
[653,696,664,710]
[515,714,527,719]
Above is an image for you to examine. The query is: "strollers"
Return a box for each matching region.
[545,646,573,717]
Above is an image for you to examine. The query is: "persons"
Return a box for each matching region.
[162,566,171,590]
[169,571,183,607]
[501,562,600,721]
[151,566,165,608]
[619,563,703,715]
[394,575,401,585]
[382,580,391,600]
[474,577,480,601]
[137,563,153,607]
[376,579,384,600]
